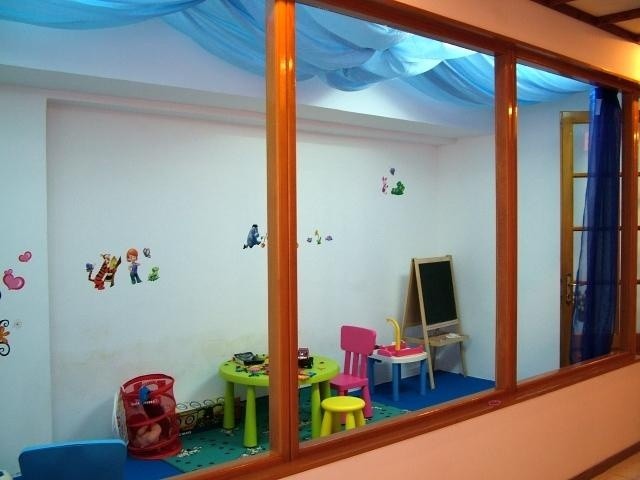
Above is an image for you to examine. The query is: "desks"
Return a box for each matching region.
[367,342,427,401]
[218,355,337,449]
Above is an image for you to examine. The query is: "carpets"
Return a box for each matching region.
[156,396,409,475]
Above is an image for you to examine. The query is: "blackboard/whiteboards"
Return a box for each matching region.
[415,255,460,331]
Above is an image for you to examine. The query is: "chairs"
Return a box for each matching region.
[330,326,378,427]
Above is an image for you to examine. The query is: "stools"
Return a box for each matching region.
[317,394,371,437]
